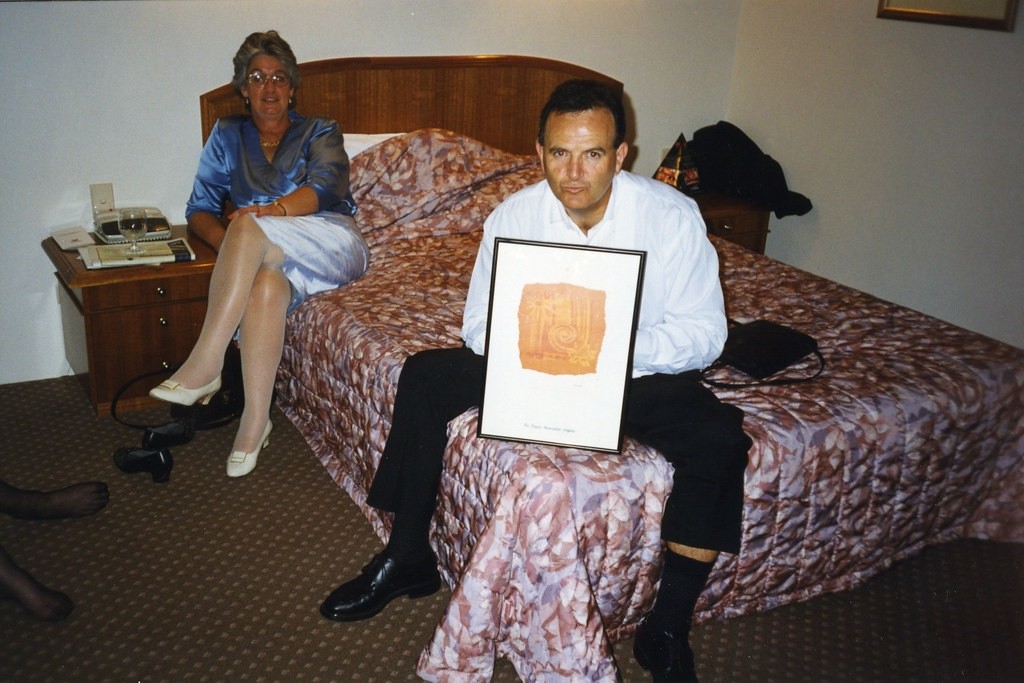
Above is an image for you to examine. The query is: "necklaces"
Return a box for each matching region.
[260,140,280,147]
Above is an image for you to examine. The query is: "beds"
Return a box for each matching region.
[200,54,1023,682]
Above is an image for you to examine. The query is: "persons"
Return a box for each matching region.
[148,29,371,477]
[320,78,745,683]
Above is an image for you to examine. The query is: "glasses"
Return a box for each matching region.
[246,71,291,86]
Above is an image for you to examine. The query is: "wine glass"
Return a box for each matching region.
[118,207,148,254]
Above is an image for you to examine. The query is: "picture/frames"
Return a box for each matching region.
[875,0,1020,33]
[475,237,648,455]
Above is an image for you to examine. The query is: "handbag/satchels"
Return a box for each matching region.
[717,320,817,379]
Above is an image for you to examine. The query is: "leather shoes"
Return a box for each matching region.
[634,623,697,683]
[320,552,441,621]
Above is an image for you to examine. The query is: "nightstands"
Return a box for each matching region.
[42,222,220,418]
[690,190,770,255]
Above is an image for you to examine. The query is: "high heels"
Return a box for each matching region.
[150,373,222,405]
[144,419,197,451]
[114,447,172,482]
[226,420,274,478]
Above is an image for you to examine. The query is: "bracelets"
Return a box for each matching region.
[273,202,286,216]
